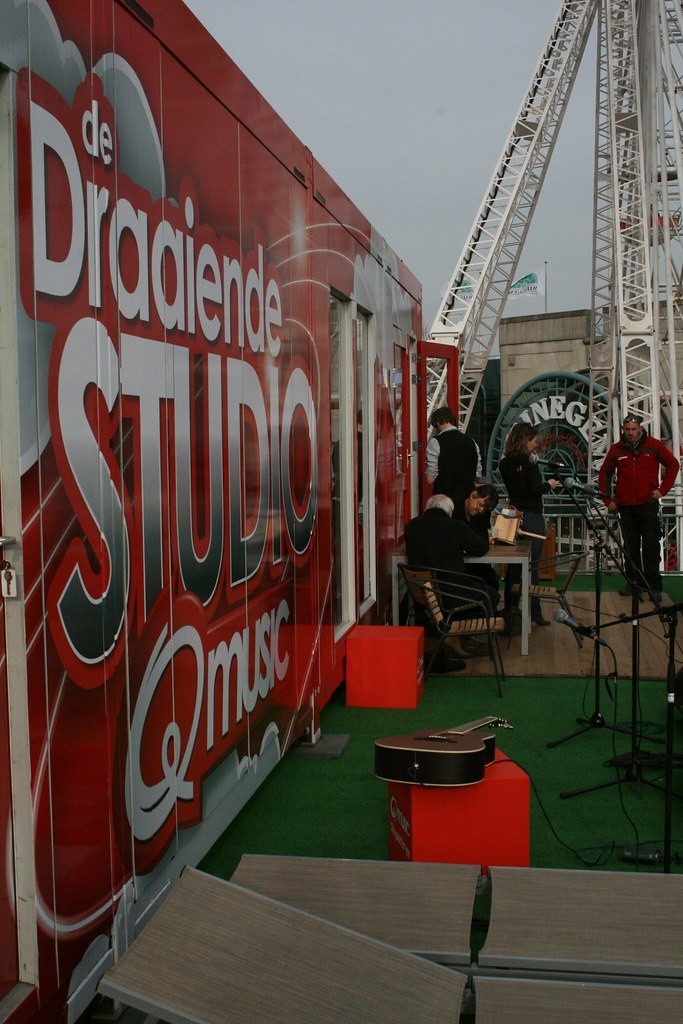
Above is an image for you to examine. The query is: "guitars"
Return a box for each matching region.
[371,713,515,789]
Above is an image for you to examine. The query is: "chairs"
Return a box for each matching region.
[397,561,490,654]
[508,550,591,647]
[411,576,506,697]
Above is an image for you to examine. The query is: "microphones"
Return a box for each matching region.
[529,455,569,469]
[564,477,606,497]
[553,609,608,647]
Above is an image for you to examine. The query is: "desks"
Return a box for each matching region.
[392,541,532,655]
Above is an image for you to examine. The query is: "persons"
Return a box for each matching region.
[499,422,561,627]
[331,409,381,601]
[599,414,680,602]
[404,484,501,659]
[425,408,483,517]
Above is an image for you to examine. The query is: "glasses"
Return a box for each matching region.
[475,496,487,513]
[623,417,639,423]
[431,419,438,429]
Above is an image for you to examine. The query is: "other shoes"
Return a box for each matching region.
[619,583,643,596]
[462,633,495,655]
[430,653,466,674]
[531,614,550,626]
[649,590,662,602]
[441,635,472,658]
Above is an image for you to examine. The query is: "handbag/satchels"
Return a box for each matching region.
[492,505,521,546]
[496,607,522,636]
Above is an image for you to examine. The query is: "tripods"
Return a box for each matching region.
[547,462,683,800]
